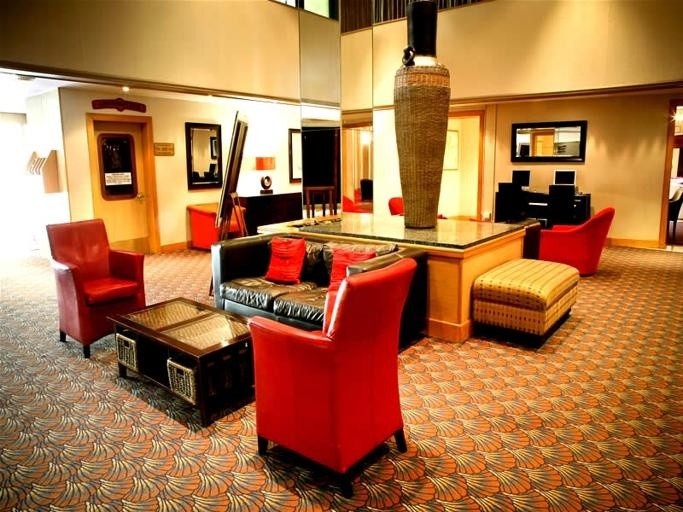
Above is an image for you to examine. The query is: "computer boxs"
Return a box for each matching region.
[528,217,537,222]
[537,218,548,228]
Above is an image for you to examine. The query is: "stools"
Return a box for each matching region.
[472,258,581,349]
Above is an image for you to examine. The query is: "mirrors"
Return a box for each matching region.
[184,121,223,190]
[287,128,303,183]
[510,119,588,162]
[341,126,373,214]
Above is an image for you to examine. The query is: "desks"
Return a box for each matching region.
[495,190,590,225]
[235,191,303,236]
[670,178,683,219]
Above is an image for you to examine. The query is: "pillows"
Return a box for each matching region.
[264,235,399,336]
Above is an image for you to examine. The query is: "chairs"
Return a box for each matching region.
[248,255,418,498]
[498,182,538,259]
[343,179,373,213]
[193,171,216,182]
[538,207,616,275]
[548,184,582,229]
[668,188,683,243]
[187,202,247,250]
[388,196,404,218]
[46,217,147,358]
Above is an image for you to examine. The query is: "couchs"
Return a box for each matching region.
[211,232,430,357]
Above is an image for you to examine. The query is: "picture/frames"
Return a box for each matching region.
[443,129,460,171]
[210,136,217,159]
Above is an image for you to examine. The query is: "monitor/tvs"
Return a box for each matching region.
[553,169,576,186]
[512,170,530,187]
